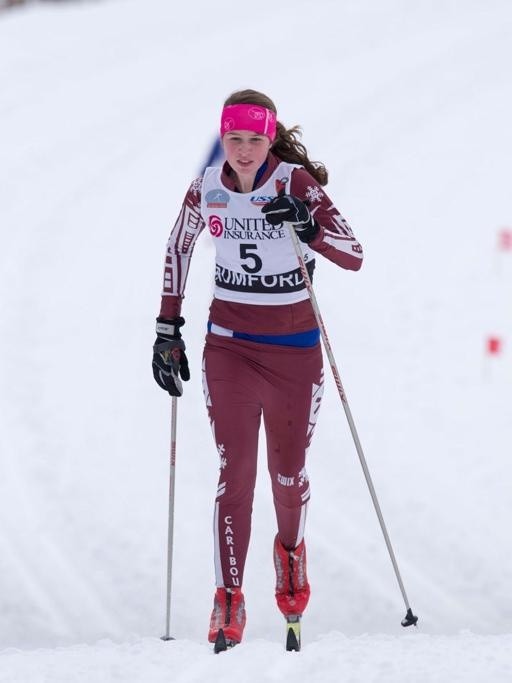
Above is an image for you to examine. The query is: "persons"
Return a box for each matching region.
[150,90,364,644]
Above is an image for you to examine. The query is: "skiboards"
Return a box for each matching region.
[214,627,299,654]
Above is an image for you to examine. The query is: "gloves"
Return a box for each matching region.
[152,318,190,397]
[261,195,320,244]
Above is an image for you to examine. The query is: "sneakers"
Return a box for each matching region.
[209,586,246,644]
[273,533,310,615]
